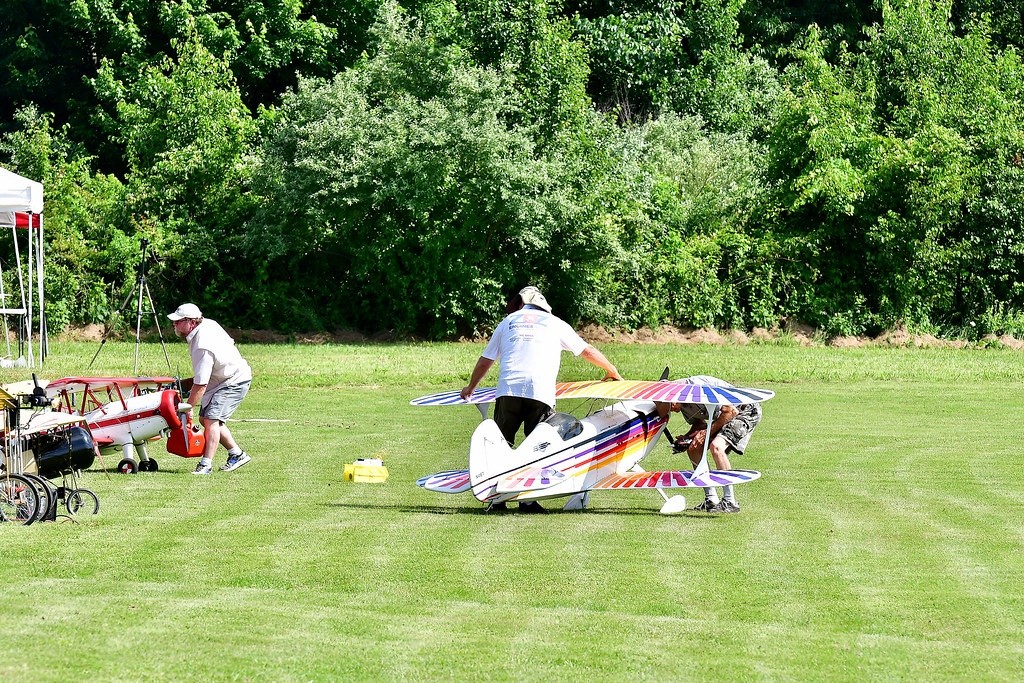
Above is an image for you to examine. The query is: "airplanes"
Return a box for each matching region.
[411,365,775,515]
[6,375,192,475]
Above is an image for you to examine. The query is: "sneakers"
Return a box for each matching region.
[219,450,251,471]
[191,463,213,474]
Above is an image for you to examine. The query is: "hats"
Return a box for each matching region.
[167,303,202,321]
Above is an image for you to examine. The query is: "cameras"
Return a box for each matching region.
[141,238,150,246]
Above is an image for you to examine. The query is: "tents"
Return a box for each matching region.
[0,165,48,369]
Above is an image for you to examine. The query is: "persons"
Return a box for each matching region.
[460,286,625,516]
[167,303,253,475]
[662,375,763,513]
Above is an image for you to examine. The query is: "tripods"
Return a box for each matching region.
[87,244,172,375]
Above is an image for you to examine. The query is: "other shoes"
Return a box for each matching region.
[519,501,547,514]
[709,498,741,514]
[693,498,719,512]
[493,505,508,512]
